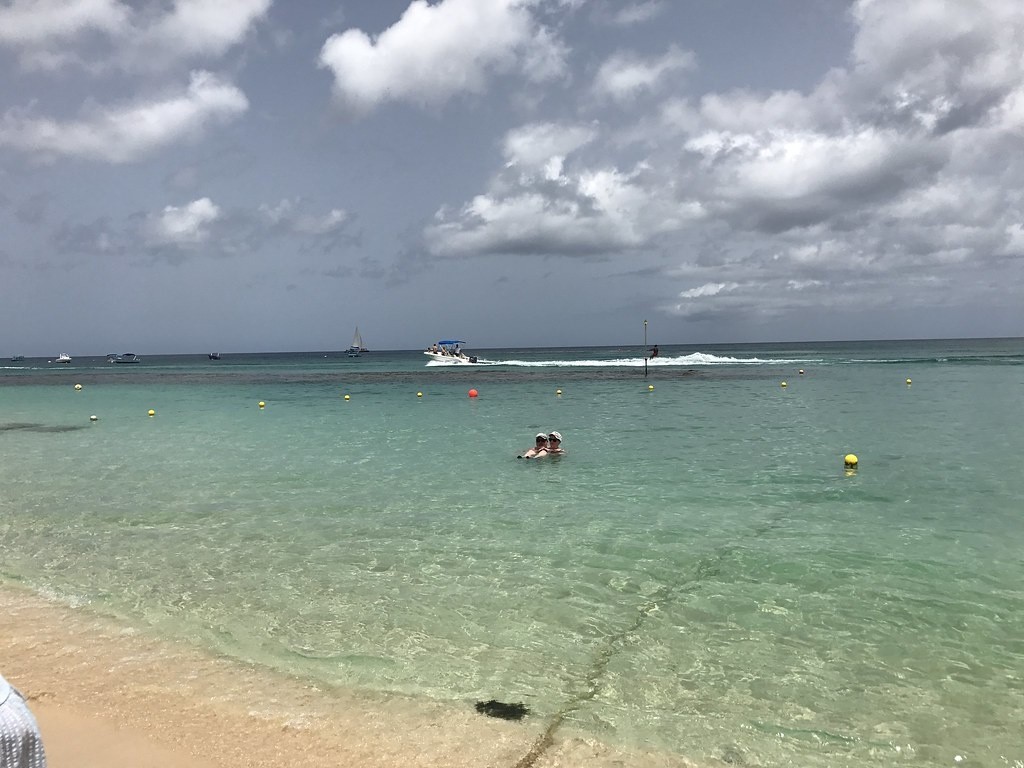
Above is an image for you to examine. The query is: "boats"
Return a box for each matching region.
[56,352,72,364]
[424,340,497,365]
[208,352,221,360]
[106,352,140,363]
[10,354,24,361]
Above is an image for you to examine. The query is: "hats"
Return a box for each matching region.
[549,431,563,444]
[535,432,548,439]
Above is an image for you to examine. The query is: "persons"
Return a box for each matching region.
[428,347,431,353]
[432,343,438,354]
[442,346,449,356]
[453,344,464,357]
[517,431,565,459]
[647,344,658,359]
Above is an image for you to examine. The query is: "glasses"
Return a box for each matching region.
[549,437,558,442]
[536,439,546,443]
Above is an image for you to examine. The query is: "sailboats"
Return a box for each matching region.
[344,325,370,357]
